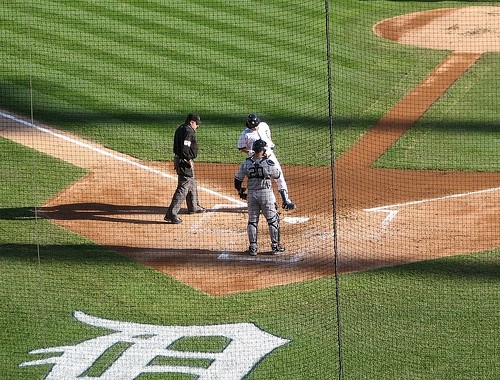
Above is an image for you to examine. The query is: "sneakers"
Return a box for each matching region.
[188,205,206,213]
[271,245,286,255]
[249,244,258,256]
[164,216,183,224]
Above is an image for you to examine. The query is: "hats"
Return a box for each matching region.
[187,113,202,124]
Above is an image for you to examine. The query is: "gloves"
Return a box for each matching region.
[282,201,294,211]
[238,187,248,200]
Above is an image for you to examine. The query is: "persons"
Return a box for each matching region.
[164,113,207,224]
[237,114,297,211]
[233,139,285,256]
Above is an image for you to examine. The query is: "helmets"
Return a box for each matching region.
[245,114,261,129]
[252,139,267,150]
